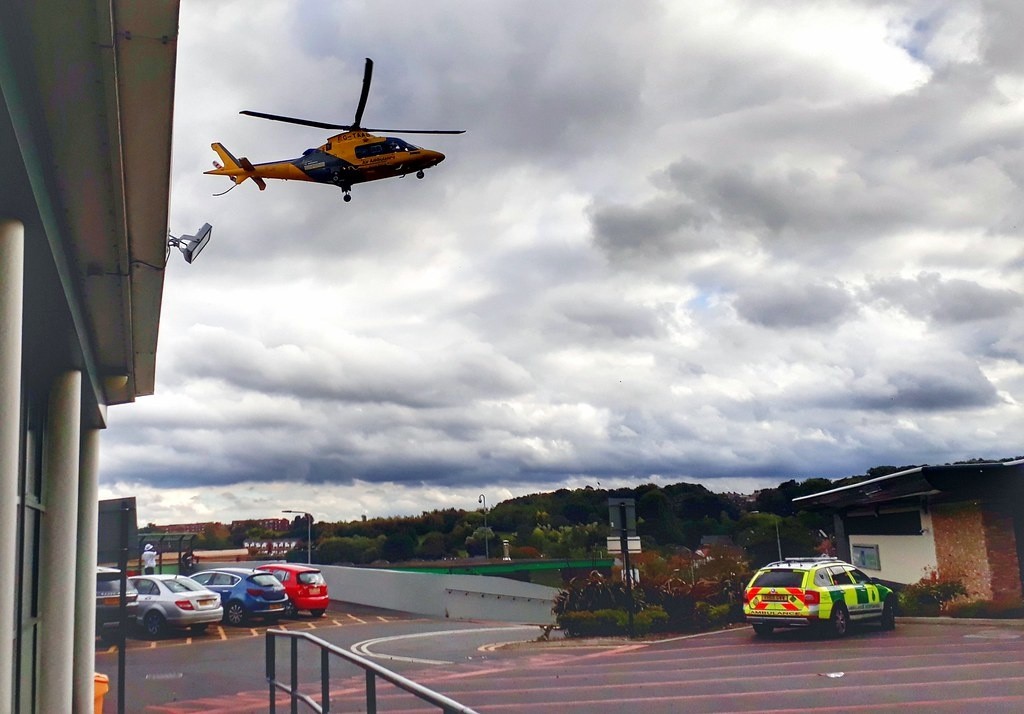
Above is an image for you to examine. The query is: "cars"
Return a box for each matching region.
[125,574,224,641]
[171,567,289,625]
[96,566,140,643]
[253,563,330,616]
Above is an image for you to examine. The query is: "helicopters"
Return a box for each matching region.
[203,57,466,200]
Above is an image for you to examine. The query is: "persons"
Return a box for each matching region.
[181,547,194,575]
[266,541,273,556]
[141,543,157,576]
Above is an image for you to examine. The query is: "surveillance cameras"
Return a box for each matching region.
[479,500,482,503]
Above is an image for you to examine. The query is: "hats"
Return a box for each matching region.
[144,544,153,550]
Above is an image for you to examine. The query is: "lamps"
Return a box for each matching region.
[169,223,212,264]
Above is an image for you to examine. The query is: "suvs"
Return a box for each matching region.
[742,553,896,638]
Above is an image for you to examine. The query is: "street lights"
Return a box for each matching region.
[478,493,488,559]
[281,510,311,564]
